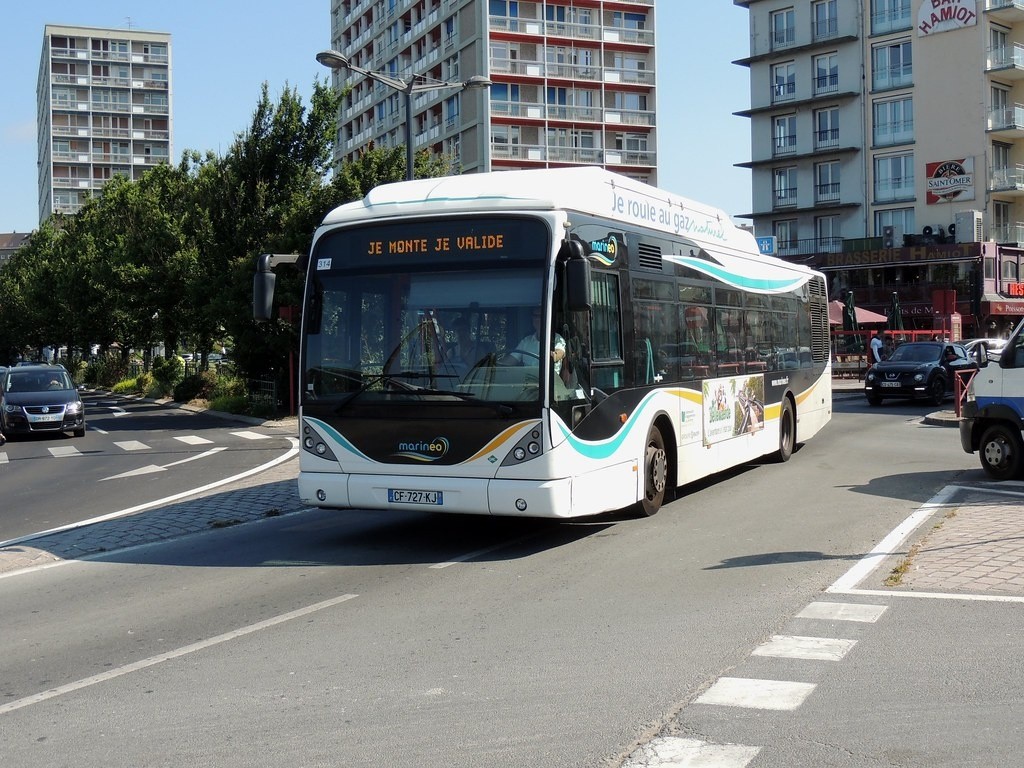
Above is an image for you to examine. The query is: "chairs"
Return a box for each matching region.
[15,377,27,391]
[440,342,498,364]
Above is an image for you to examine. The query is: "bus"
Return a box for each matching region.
[252,166,833,520]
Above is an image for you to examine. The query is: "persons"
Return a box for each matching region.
[869,325,892,364]
[446,320,485,366]
[499,308,566,375]
[1002,322,1014,340]
[46,375,63,388]
[931,334,937,341]
[988,322,997,339]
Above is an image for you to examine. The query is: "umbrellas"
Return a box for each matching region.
[829,299,887,352]
[842,292,862,348]
[891,292,907,342]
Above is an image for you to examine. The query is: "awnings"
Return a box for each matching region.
[982,302,1024,319]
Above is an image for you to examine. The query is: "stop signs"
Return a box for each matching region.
[685,307,704,329]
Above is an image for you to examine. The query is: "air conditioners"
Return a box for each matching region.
[883,224,902,238]
[955,209,984,244]
[922,224,943,237]
[883,237,903,249]
[943,223,955,238]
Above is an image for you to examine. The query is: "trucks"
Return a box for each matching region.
[959,318,1024,482]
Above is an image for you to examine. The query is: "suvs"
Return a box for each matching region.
[0,363,85,441]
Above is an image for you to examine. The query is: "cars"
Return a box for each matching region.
[865,342,980,408]
[952,338,1009,362]
[182,353,201,362]
[16,362,51,380]
[766,347,812,372]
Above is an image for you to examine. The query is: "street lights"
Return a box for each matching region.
[316,49,493,181]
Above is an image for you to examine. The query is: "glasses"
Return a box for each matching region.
[532,314,544,319]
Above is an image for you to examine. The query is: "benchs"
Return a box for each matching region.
[831,363,867,380]
